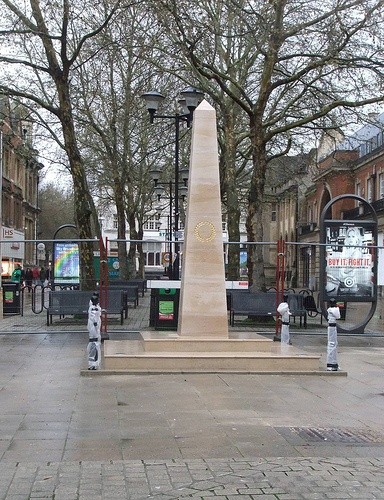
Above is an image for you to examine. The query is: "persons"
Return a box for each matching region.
[46,268,49,277]
[11,264,22,284]
[40,267,48,285]
[338,227,362,292]
[24,268,34,293]
[32,266,39,283]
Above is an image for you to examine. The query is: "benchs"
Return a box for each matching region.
[46,278,148,325]
[227,289,323,328]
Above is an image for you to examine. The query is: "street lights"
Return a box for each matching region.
[140,87,206,281]
[37,243,45,272]
